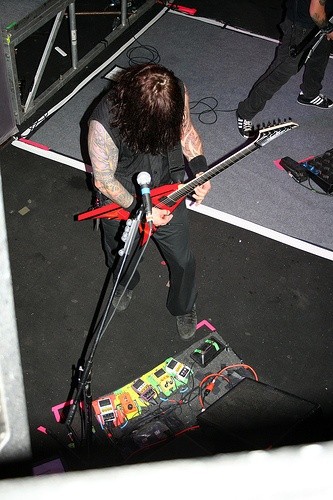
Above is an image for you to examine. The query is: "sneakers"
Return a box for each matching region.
[176,305,197,339]
[112,284,133,310]
[297,92,333,109]
[238,115,253,138]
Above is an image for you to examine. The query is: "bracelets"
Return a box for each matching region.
[188,155,208,176]
[124,197,138,213]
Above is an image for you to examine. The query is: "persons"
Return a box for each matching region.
[87,63,211,339]
[236,0,333,138]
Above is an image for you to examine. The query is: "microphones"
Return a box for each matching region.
[137,171,153,223]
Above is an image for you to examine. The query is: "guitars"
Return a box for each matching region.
[74,117,299,249]
